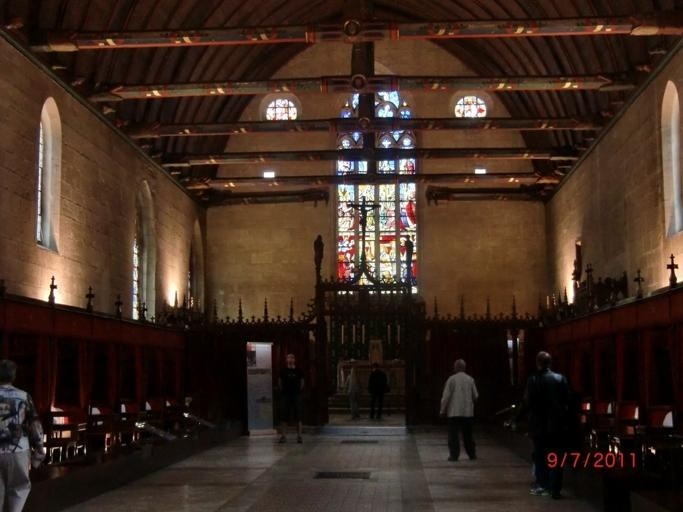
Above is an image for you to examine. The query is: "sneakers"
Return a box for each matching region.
[529,487,552,496]
[448,454,477,461]
[279,436,302,443]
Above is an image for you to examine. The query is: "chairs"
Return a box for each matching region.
[560,396,681,491]
[44,399,199,466]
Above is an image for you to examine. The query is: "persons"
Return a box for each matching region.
[367,363,387,420]
[277,354,305,443]
[314,236,324,267]
[515,350,575,496]
[0,358,46,512]
[439,359,480,462]
[405,236,414,265]
[345,367,362,420]
